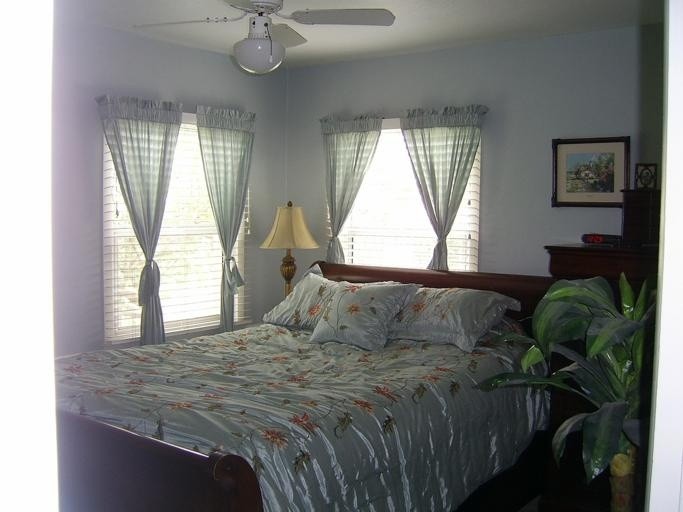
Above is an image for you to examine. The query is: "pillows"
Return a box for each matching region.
[256,261,529,352]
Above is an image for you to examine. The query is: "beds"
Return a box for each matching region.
[56,261,559,512]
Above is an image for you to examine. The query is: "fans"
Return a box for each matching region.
[130,0,397,47]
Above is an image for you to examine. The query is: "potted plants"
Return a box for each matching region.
[471,272,656,511]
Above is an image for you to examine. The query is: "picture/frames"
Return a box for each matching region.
[633,163,657,189]
[551,136,631,209]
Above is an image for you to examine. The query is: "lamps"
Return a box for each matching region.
[232,40,286,73]
[259,202,319,299]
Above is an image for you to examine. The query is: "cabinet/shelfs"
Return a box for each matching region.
[543,187,659,295]
